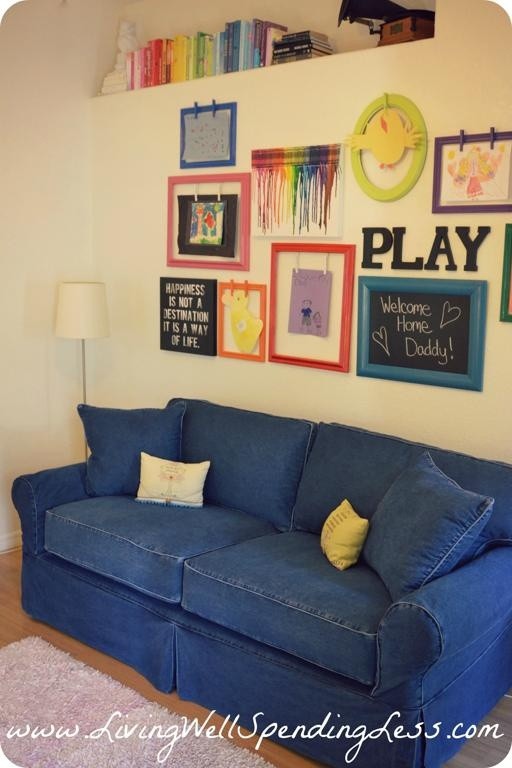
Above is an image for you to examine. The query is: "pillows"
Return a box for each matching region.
[318,496,371,573]
[72,399,188,502]
[135,447,213,511]
[361,447,495,608]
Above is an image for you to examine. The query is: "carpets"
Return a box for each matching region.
[0,632,283,768]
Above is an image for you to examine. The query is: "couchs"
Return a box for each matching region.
[7,393,511,768]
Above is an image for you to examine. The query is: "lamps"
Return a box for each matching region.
[54,279,112,468]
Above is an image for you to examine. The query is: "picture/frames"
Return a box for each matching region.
[264,241,356,375]
[213,279,269,364]
[497,221,512,325]
[352,273,489,395]
[163,171,253,273]
[431,128,512,215]
[342,93,432,201]
